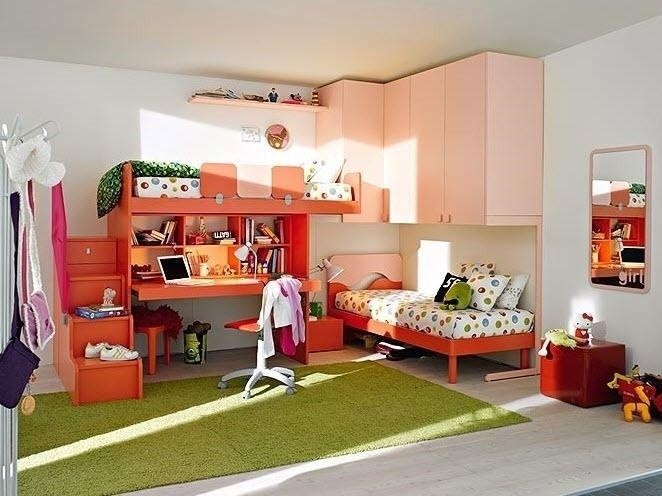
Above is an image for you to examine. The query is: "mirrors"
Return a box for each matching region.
[587,144,651,293]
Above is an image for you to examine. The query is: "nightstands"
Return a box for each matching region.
[308,315,343,353]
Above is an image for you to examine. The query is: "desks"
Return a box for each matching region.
[539,337,626,408]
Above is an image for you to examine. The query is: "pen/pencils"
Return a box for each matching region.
[198,255,208,263]
[591,242,601,253]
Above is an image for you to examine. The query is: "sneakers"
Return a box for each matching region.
[85,342,109,358]
[100,344,139,360]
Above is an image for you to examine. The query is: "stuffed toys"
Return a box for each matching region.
[616,378,657,423]
[537,328,587,359]
[571,314,597,348]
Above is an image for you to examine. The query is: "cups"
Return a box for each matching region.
[197,262,211,277]
[590,252,599,268]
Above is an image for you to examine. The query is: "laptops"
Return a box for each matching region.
[619,246,645,266]
[157,255,213,286]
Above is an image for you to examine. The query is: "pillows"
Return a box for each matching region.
[433,261,529,312]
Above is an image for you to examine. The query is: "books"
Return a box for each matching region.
[244,216,285,274]
[132,218,179,246]
[611,221,631,241]
[90,304,125,311]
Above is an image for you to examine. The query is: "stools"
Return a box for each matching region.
[134,324,171,375]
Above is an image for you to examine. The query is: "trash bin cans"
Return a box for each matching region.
[183,330,207,365]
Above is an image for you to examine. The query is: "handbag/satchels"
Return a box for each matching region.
[20,290,56,353]
[0,336,41,408]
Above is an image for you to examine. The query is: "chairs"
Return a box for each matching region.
[217,278,307,399]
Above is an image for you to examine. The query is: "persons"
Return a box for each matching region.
[268,88,278,102]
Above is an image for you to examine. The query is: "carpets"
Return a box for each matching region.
[563,468,662,496]
[16,358,532,496]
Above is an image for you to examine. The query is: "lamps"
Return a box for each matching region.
[309,279,322,319]
[309,257,343,320]
[233,241,258,280]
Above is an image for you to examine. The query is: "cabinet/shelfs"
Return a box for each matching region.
[54,163,360,409]
[591,217,645,265]
[314,53,543,227]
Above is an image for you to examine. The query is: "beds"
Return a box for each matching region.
[328,253,534,384]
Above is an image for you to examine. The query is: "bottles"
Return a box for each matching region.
[199,216,207,238]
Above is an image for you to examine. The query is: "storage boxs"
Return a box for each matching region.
[592,180,630,207]
[627,192,646,208]
[302,182,352,202]
[132,176,203,199]
